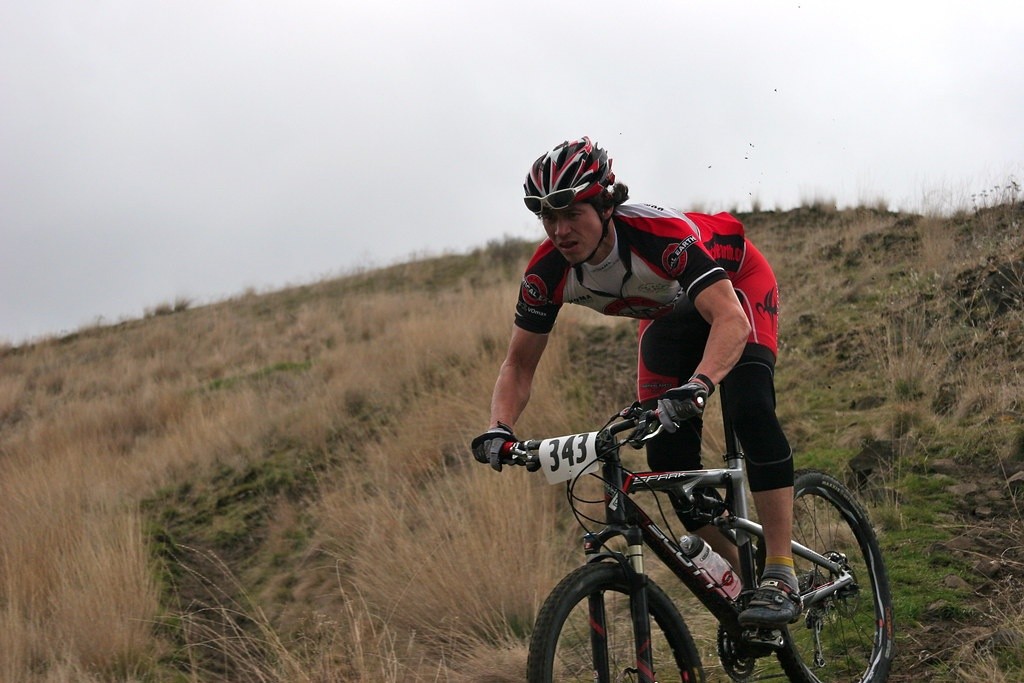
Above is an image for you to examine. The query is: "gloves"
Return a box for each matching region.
[470,419,517,472]
[653,374,715,434]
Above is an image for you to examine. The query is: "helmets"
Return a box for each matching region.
[525,140,613,214]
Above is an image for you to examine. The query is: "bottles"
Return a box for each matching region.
[680,534,742,604]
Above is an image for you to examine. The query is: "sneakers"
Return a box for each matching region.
[739,578,803,628]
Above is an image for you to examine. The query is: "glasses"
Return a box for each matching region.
[525,178,597,215]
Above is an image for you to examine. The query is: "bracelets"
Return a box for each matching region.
[688,373,715,397]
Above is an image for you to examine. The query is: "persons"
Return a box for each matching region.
[471,136,800,626]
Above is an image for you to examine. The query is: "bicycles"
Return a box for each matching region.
[497,395,895,683]
